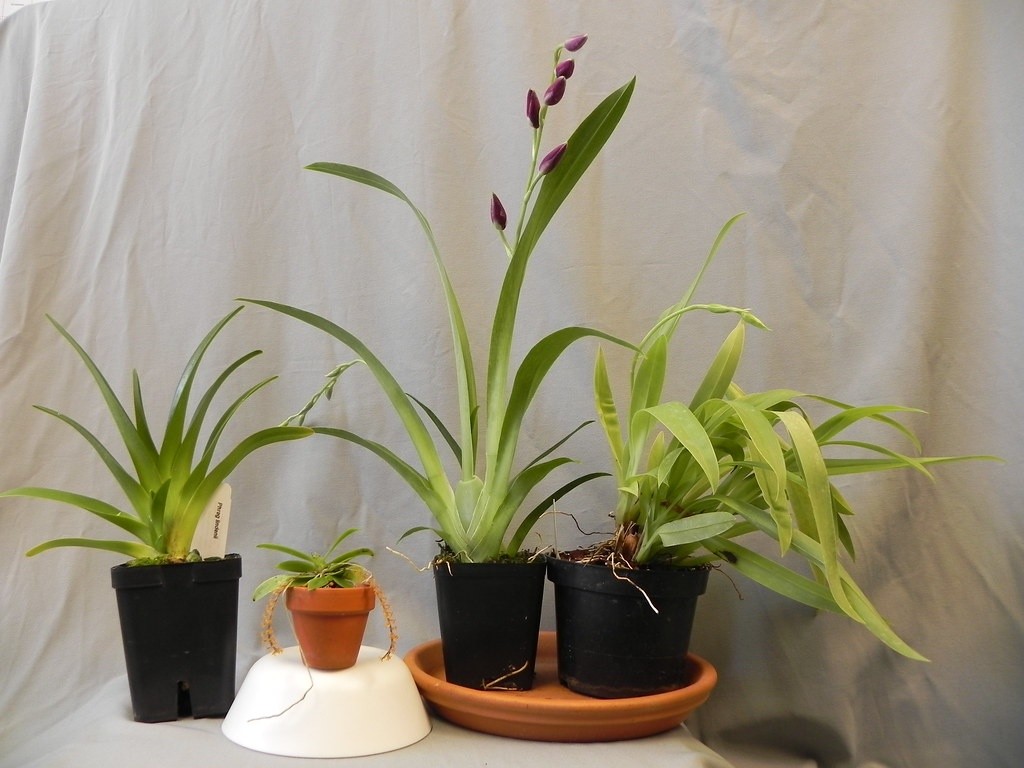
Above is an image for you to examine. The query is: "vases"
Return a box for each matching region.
[430,547,549,693]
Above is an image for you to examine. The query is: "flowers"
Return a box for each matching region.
[304,16,634,559]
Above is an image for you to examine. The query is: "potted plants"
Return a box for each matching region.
[547,210,1005,700]
[254,528,399,669]
[7,300,315,724]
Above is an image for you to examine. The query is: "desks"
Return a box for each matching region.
[18,643,729,768]
